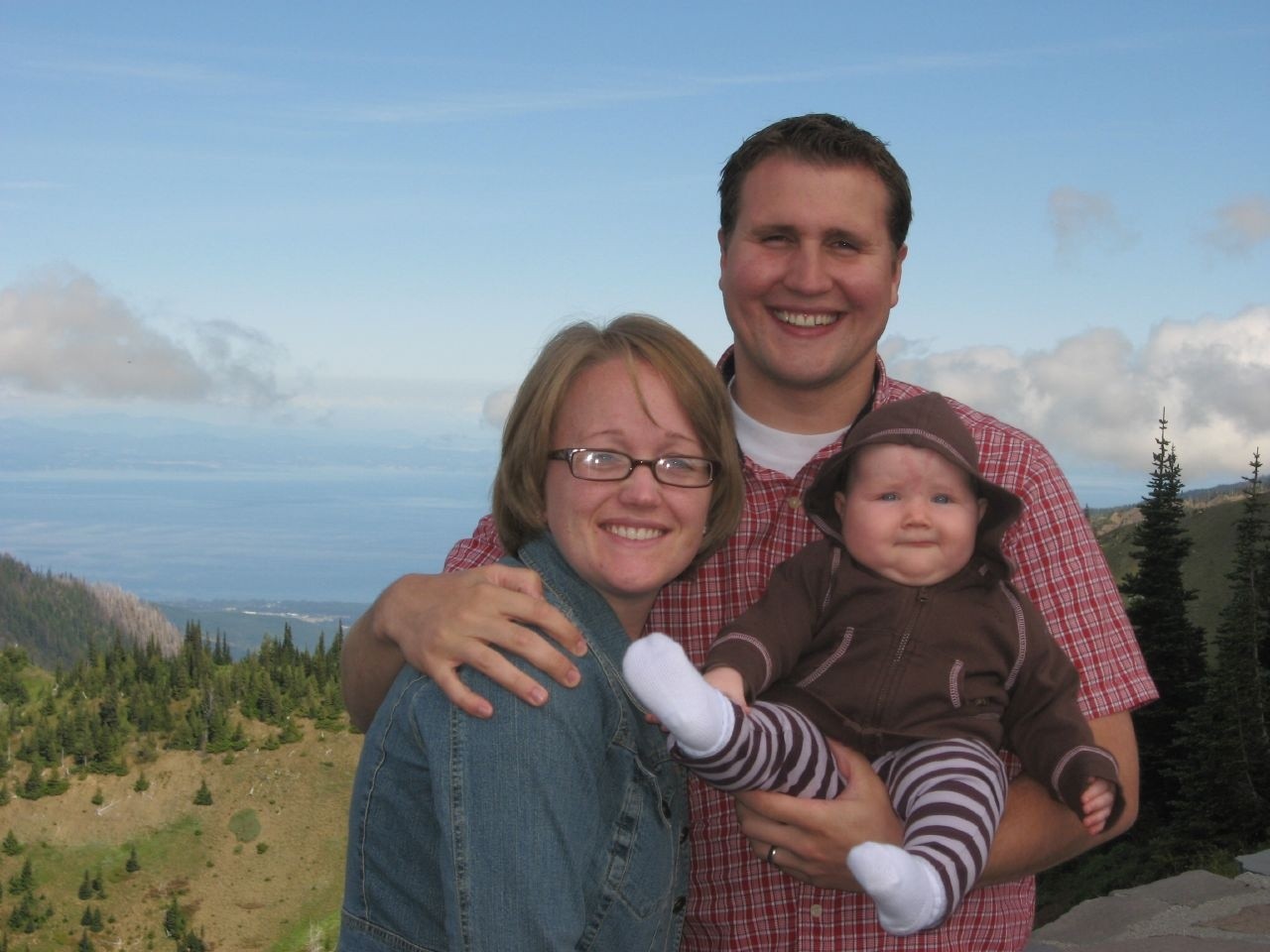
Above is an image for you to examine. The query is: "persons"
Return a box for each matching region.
[621,391,1126,936]
[338,314,744,952]
[339,112,1160,952]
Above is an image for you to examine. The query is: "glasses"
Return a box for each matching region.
[548,448,721,488]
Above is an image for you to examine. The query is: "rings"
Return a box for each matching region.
[767,845,778,865]
